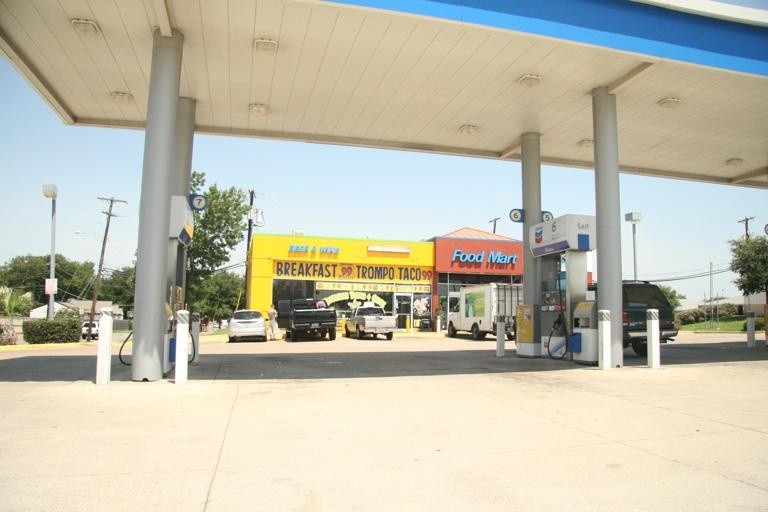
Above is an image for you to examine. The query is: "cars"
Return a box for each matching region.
[81,321,99,339]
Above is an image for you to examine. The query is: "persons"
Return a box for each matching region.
[267,305,278,340]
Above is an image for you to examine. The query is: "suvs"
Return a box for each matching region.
[586,279,678,356]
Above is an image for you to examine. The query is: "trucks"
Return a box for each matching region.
[448,284,524,340]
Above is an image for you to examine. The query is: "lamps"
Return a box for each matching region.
[111,92,133,105]
[71,19,100,37]
[727,159,743,165]
[248,103,268,117]
[254,39,277,59]
[457,124,479,136]
[515,74,542,88]
[657,97,681,107]
[578,139,594,147]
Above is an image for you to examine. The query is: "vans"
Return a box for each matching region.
[228,309,269,341]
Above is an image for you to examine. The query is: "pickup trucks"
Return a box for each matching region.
[345,306,399,340]
[278,299,337,341]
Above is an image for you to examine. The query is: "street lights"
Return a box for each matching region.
[43,184,58,320]
[625,212,642,281]
